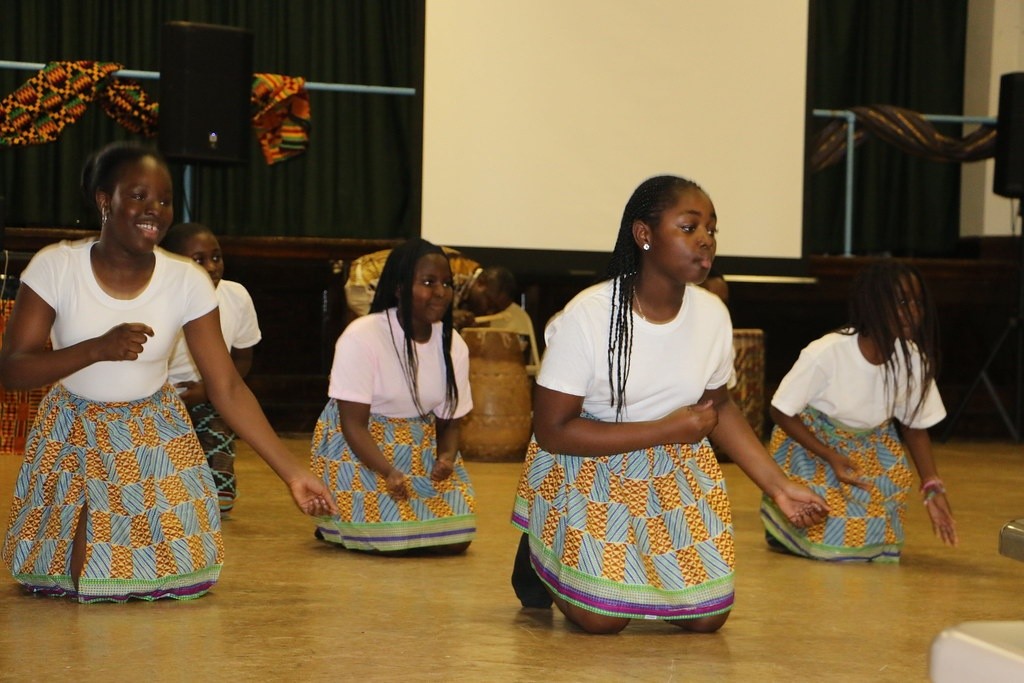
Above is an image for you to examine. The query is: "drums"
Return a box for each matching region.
[459,326,534,463]
[710,329,765,463]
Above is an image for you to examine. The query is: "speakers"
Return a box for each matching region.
[157,22,252,169]
[993,71,1024,197]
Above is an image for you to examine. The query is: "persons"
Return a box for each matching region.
[344,245,519,329]
[699,268,730,307]
[759,263,956,563]
[0,144,340,604]
[511,175,834,634]
[156,222,261,517]
[309,238,479,557]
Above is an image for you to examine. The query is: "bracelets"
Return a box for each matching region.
[922,478,947,507]
